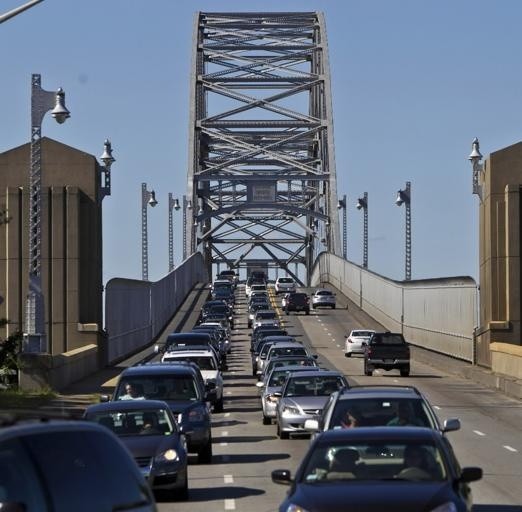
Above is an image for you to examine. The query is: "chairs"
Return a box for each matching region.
[331,450,369,479]
[293,384,305,393]
[98,417,114,431]
[135,383,144,392]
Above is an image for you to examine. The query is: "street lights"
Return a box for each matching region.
[141,181,158,281]
[395,181,412,283]
[21,72,70,353]
[336,195,348,258]
[183,194,192,259]
[168,192,180,275]
[356,190,369,272]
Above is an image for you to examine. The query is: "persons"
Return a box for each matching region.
[119,382,144,400]
[386,403,420,426]
[342,407,362,427]
[403,445,431,476]
[276,376,285,386]
